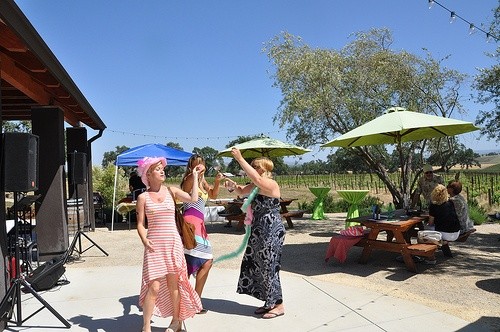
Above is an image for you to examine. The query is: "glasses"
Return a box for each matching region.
[425,171,433,174]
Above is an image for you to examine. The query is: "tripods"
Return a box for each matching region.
[0,192,71,329]
[63,184,109,266]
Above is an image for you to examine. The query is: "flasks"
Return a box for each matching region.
[373,203,380,220]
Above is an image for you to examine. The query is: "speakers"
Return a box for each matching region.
[67,153,87,184]
[24,255,65,292]
[2,132,38,193]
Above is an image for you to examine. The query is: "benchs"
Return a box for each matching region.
[309,229,477,255]
[225,211,306,221]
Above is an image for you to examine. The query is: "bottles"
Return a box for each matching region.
[387,202,393,221]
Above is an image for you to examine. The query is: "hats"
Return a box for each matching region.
[423,164,432,173]
[137,156,167,189]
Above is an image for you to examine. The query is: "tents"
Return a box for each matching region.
[111,143,197,231]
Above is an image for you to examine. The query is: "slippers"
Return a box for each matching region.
[254,305,274,315]
[262,311,285,319]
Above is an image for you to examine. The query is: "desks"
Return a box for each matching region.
[347,209,454,273]
[114,201,226,228]
[227,200,297,233]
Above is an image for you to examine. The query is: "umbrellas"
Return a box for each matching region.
[318,106,479,206]
[217,137,313,158]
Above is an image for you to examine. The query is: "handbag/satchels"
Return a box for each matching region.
[418,230,443,247]
[342,225,364,237]
[167,187,197,250]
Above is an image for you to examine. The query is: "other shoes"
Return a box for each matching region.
[165,319,182,332]
[420,259,437,264]
[442,247,452,258]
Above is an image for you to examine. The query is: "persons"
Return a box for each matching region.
[419,179,468,264]
[177,153,223,314]
[128,172,147,200]
[225,147,286,318]
[418,164,445,204]
[135,157,203,332]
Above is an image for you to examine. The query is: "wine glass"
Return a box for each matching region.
[227,181,236,203]
[213,159,226,179]
